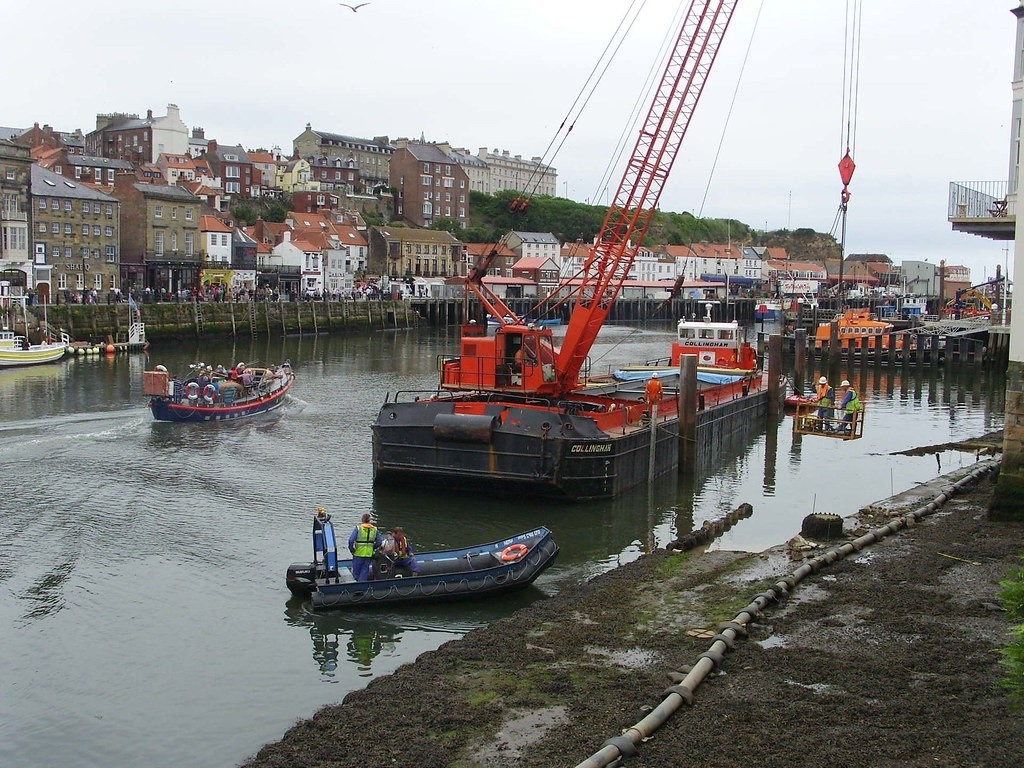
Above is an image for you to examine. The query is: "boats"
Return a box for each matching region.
[142,360,296,424]
[285,504,562,612]
[782,391,819,406]
[775,308,946,369]
[486,311,563,326]
[369,300,789,501]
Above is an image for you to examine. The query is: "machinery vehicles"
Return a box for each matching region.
[945,289,994,320]
[433,0,742,432]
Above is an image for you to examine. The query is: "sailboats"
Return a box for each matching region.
[0,289,67,369]
[65,293,148,356]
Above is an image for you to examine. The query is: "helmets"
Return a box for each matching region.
[841,381,850,386]
[819,376,827,384]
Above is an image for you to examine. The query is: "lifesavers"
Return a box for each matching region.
[501,543,528,561]
[839,326,883,336]
[716,357,729,369]
[153,364,169,382]
[185,382,200,400]
[203,384,216,402]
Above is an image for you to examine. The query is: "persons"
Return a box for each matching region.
[515,345,529,372]
[27,284,428,306]
[348,514,382,581]
[645,373,663,417]
[198,362,255,396]
[817,377,834,430]
[381,527,420,577]
[835,381,860,434]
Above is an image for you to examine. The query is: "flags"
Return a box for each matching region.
[129,293,140,316]
